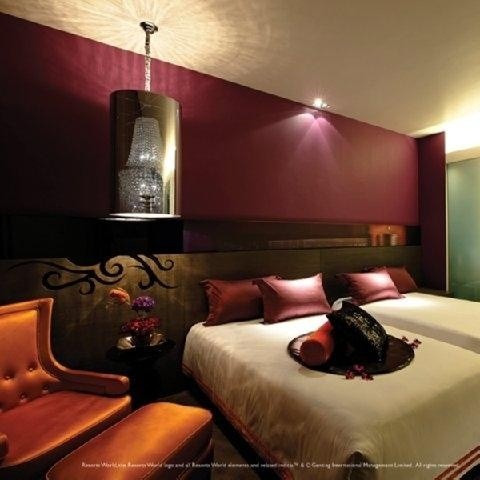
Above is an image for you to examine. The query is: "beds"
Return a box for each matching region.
[181,314,480,480]
[331,293,480,354]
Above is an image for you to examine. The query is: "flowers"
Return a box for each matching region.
[108,287,161,338]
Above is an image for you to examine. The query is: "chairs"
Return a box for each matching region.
[0,298,132,480]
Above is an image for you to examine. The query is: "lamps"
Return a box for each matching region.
[106,21,182,218]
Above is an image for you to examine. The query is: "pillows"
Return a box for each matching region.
[199,265,419,326]
[299,301,388,366]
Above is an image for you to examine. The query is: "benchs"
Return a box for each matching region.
[44,401,216,480]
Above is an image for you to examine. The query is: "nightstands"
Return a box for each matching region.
[408,284,454,298]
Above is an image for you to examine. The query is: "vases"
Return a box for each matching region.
[132,334,150,348]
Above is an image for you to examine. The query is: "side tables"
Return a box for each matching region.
[105,339,176,408]
